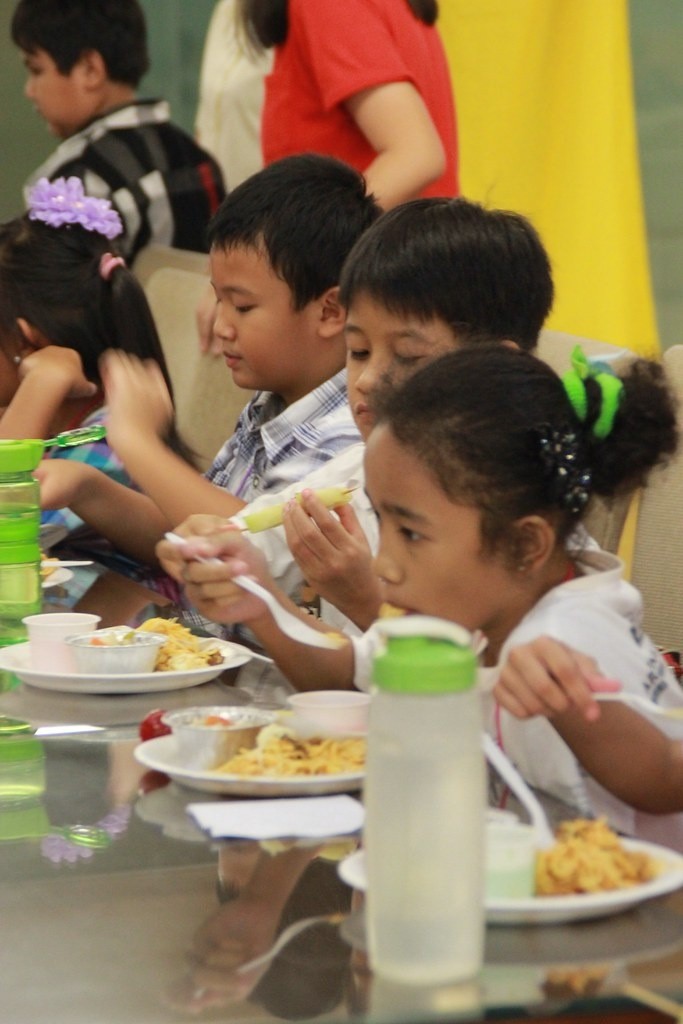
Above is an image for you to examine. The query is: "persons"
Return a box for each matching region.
[0,1,682,837]
[155,196,602,661]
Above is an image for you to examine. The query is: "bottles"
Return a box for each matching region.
[0,437,44,648]
[364,615,489,989]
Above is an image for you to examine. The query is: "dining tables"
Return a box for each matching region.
[0,553,683,1024]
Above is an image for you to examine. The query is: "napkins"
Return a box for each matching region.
[182,792,366,842]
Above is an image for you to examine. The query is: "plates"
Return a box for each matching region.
[1,642,249,694]
[336,829,683,924]
[133,733,362,796]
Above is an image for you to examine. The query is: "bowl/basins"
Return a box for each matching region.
[287,689,371,737]
[64,630,168,674]
[164,704,277,770]
[21,612,101,670]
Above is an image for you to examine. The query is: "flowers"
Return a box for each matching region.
[27,174,125,240]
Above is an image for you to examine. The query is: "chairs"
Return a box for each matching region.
[113,241,683,676]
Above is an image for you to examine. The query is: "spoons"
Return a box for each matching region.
[161,534,341,650]
